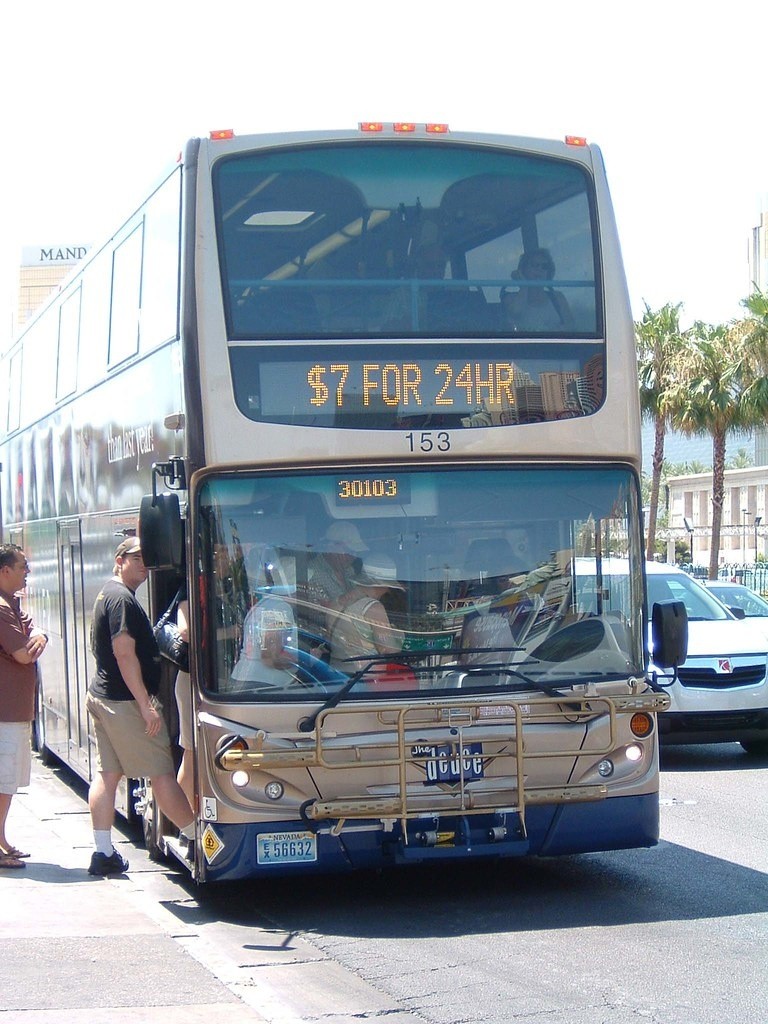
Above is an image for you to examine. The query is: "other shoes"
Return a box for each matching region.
[179,831,187,847]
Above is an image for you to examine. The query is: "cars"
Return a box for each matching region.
[567,557,768,758]
[665,579,767,622]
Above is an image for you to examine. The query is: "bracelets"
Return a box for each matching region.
[43,633,48,643]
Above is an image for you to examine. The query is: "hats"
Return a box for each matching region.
[326,523,369,555]
[270,556,315,595]
[347,552,405,592]
[115,538,142,560]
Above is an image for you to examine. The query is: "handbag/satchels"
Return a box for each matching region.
[153,590,189,668]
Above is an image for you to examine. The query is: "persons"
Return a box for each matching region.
[173,600,240,846]
[308,521,371,681]
[85,536,196,875]
[502,249,574,332]
[323,553,404,685]
[461,555,565,643]
[228,556,316,691]
[0,543,47,868]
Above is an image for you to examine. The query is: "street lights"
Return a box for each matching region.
[755,516,763,573]
[681,516,695,566]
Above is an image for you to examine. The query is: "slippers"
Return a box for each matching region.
[0,851,27,868]
[4,847,30,858]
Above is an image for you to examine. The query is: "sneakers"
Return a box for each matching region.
[186,840,194,862]
[88,845,129,874]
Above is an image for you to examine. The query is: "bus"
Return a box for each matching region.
[1,122,695,893]
